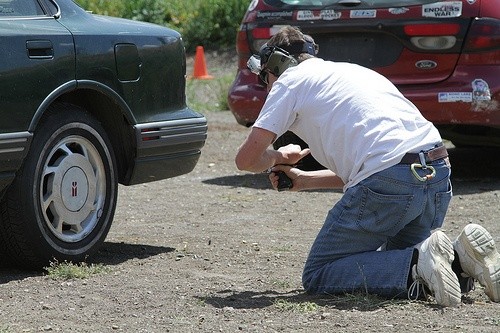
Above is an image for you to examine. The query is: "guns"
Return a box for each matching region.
[261,163,298,192]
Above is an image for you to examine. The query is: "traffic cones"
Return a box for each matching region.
[189,45,213,79]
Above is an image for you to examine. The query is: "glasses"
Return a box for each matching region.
[260,66,270,85]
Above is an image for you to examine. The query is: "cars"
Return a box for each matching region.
[1,0,209,264]
[227,0,500,153]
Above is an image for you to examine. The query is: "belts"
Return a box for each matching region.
[400,145,449,166]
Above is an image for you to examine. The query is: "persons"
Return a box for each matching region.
[235,25,500,308]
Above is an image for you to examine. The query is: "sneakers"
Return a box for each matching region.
[408,231,461,307]
[454,223,500,303]
